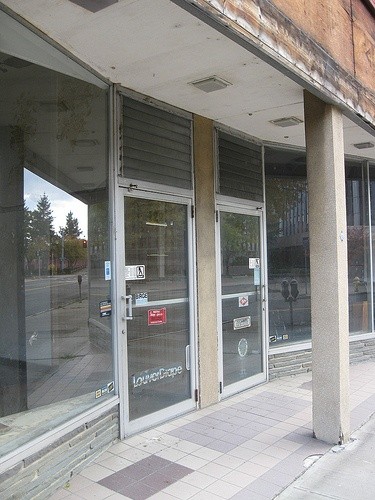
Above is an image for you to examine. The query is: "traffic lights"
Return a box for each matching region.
[83,240,87,247]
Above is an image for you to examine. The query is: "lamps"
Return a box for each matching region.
[68,0,118,14]
[353,142,374,150]
[269,116,304,128]
[191,74,232,94]
